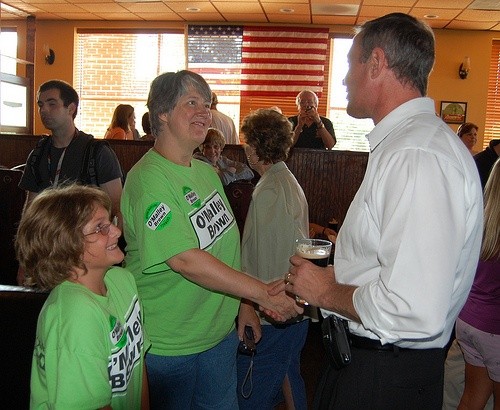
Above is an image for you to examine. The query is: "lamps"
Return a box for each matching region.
[458,56,471,80]
[42,41,55,65]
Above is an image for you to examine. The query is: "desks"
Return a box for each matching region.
[0,135,370,227]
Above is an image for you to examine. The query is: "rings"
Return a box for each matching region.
[286,273,292,280]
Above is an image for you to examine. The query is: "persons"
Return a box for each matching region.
[455,159,500,410]
[209,92,239,145]
[105,104,153,140]
[121,70,304,410]
[238,107,311,410]
[288,90,336,150]
[192,128,255,185]
[307,223,338,238]
[473,138,500,189]
[16,185,152,410]
[260,12,485,410]
[18,80,123,217]
[457,123,478,152]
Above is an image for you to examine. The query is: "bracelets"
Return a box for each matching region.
[318,119,322,125]
[321,228,326,235]
[318,123,325,130]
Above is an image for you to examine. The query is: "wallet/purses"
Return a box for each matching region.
[313,315,354,369]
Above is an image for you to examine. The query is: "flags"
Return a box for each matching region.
[182,23,329,98]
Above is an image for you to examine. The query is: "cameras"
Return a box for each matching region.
[240,326,257,354]
[306,106,312,111]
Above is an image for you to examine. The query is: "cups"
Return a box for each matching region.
[295,239,333,305]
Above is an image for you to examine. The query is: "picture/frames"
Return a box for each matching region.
[439,99,468,126]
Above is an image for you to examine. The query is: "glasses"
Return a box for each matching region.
[82,216,119,236]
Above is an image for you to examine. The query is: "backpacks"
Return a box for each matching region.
[227,178,256,233]
